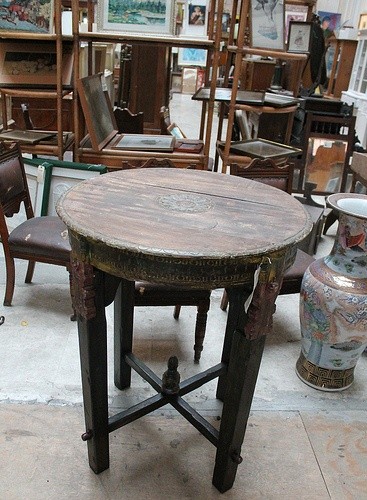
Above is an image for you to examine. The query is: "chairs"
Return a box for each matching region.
[0,149,71,306]
[135,281,211,364]
[220,156,315,311]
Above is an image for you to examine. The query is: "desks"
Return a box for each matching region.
[56,168,313,492]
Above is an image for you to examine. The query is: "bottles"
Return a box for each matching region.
[294,191,366,393]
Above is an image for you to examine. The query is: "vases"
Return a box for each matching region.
[295,192,367,392]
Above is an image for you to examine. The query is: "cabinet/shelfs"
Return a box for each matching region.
[0,0,367,195]
[323,38,358,99]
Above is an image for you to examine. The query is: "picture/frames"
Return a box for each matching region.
[177,47,208,67]
[208,12,229,32]
[0,39,75,90]
[0,129,57,144]
[218,137,304,160]
[248,0,281,47]
[358,13,367,30]
[181,66,199,95]
[264,92,307,109]
[76,71,120,152]
[167,122,189,139]
[97,0,175,38]
[286,21,315,54]
[111,133,175,152]
[191,86,266,106]
